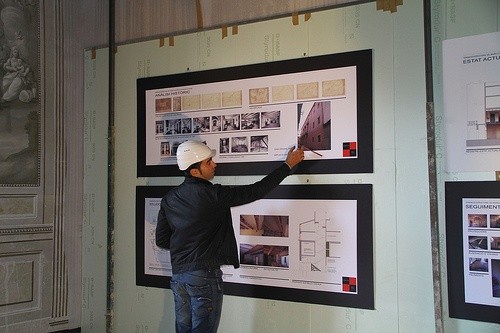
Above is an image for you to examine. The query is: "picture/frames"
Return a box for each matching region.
[136,49,373,177]
[445,181,500,325]
[136,184,373,310]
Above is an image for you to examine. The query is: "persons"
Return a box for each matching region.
[156,140,305,333]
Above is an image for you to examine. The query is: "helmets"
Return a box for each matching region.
[176,141,216,170]
